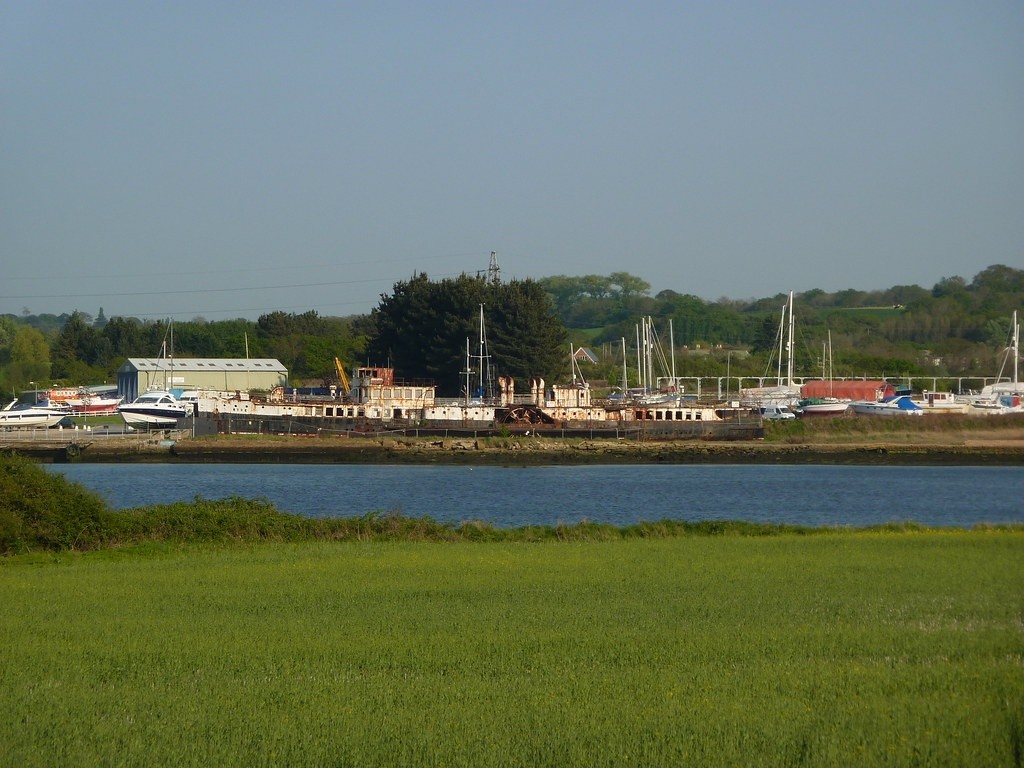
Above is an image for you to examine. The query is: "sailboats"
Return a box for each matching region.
[734,288,804,410]
[801,330,849,413]
[970,309,1024,412]
[601,315,685,404]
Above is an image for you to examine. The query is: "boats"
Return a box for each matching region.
[64,395,124,414]
[175,365,642,437]
[176,390,197,414]
[911,392,968,408]
[0,397,67,429]
[849,394,923,416]
[117,312,185,429]
[31,399,68,413]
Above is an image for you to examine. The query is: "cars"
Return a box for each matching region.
[471,401,484,404]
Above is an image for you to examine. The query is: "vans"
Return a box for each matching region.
[763,404,795,420]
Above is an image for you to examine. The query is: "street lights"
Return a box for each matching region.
[79,386,87,421]
[30,382,37,405]
